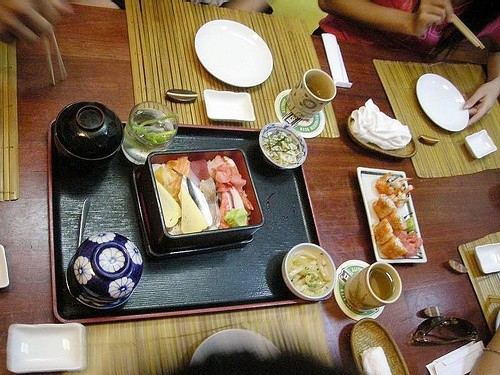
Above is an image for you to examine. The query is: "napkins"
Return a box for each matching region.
[350,98,413,151]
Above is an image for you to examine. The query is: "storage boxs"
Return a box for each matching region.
[140,148,265,253]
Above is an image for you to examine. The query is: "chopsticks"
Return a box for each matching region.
[43,31,68,86]
[451,15,486,51]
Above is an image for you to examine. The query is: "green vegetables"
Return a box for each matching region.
[126,112,178,149]
[261,130,291,153]
[303,267,329,296]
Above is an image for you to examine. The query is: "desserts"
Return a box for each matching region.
[374,173,423,260]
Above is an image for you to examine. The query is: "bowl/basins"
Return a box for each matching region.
[145,146,264,255]
[55,101,124,166]
[66,231,143,314]
[258,122,308,171]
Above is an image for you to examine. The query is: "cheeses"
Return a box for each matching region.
[156,180,208,233]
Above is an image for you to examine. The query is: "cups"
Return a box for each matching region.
[121,101,178,166]
[345,261,403,312]
[286,68,338,120]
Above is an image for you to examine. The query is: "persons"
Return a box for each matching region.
[312,0,500,126]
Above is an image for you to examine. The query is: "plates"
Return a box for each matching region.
[416,72,468,132]
[1,246,9,289]
[194,19,273,87]
[357,167,428,266]
[204,88,256,124]
[473,243,500,275]
[183,328,283,365]
[6,322,87,373]
[280,241,336,306]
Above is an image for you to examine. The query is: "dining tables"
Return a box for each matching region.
[0,8,500,375]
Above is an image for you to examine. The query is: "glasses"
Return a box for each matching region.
[413,315,480,345]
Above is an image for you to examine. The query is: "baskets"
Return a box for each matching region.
[351,320,410,375]
[347,114,417,160]
